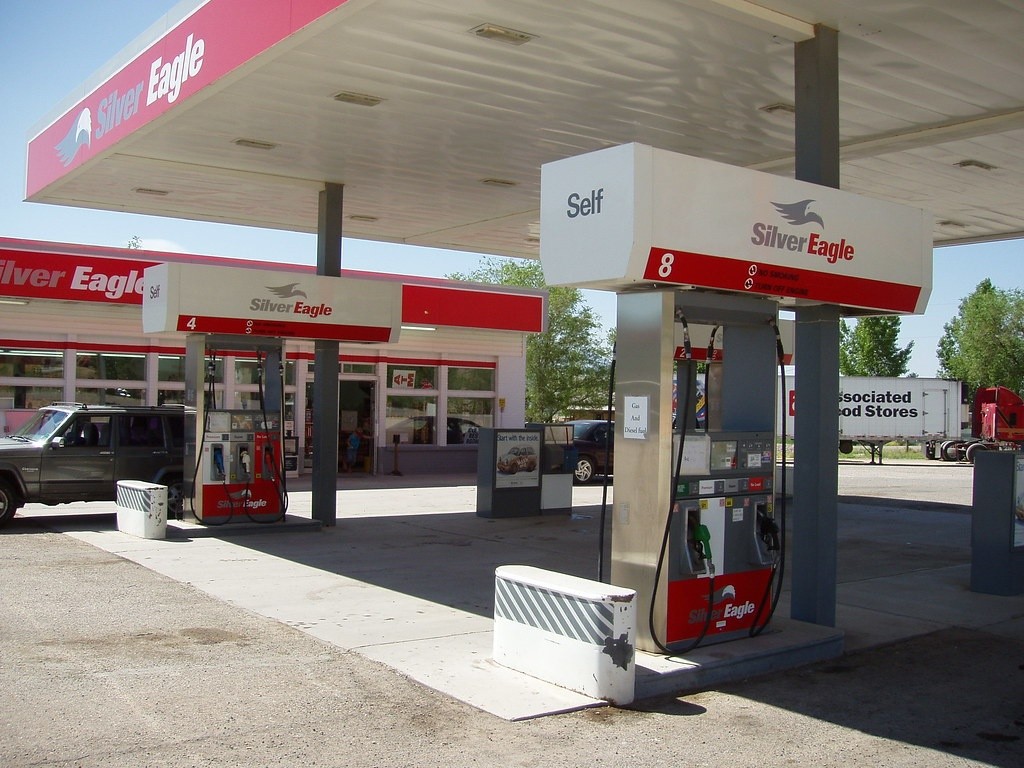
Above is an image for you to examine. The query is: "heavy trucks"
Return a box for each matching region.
[670,375,1024,462]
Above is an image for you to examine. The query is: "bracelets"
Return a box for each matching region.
[368,436,370,440]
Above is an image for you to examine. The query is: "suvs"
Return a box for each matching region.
[0,401,198,524]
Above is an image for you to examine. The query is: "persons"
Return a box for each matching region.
[345,426,375,472]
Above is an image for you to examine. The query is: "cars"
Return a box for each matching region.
[565,420,615,483]
[378,414,487,446]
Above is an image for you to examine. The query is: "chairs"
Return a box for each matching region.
[82,423,100,447]
